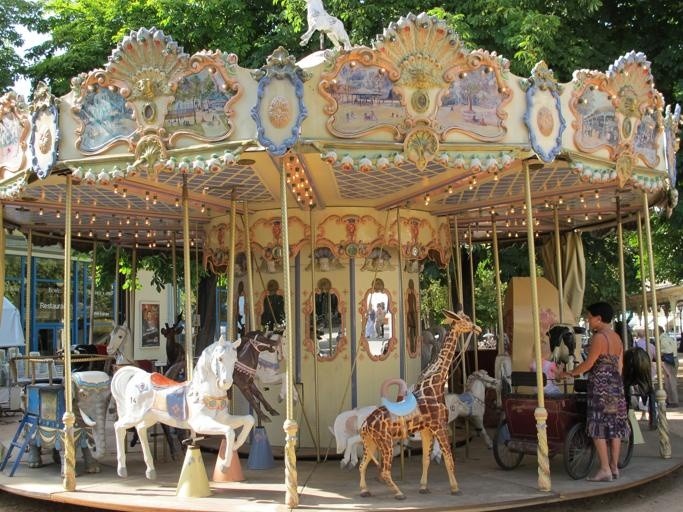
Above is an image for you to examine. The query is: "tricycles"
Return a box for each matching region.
[493,371,634,480]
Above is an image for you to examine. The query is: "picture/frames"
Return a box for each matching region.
[140,301,161,349]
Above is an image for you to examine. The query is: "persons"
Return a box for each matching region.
[527,339,568,397]
[565,299,628,482]
[653,325,680,408]
[633,329,664,421]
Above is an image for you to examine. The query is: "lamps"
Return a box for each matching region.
[424,171,602,239]
[39,183,206,248]
[287,156,313,206]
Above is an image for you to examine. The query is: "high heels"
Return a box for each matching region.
[586,474,619,481]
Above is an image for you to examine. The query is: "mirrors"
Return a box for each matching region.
[235,281,423,364]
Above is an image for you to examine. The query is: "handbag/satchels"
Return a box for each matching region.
[662,353,674,364]
